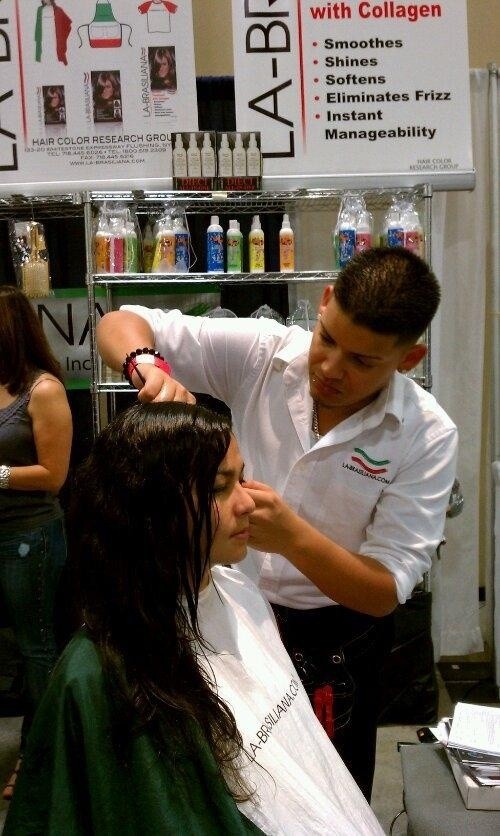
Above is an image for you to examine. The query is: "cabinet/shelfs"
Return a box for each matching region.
[80,181,434,595]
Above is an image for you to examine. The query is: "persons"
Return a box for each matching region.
[94,247,458,806]
[1,282,73,800]
[93,71,119,108]
[152,47,175,88]
[44,86,64,111]
[1,402,386,836]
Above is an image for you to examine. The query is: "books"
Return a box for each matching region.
[431,696,500,786]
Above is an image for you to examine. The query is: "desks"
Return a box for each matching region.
[366,715,497,836]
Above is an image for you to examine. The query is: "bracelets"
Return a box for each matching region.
[123,347,173,388]
[2,464,15,489]
[127,354,171,379]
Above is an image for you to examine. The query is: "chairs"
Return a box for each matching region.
[1,726,24,834]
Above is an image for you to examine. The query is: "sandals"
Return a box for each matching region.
[2,756,23,800]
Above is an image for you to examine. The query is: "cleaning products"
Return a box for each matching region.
[88,212,192,273]
[279,214,295,273]
[248,215,265,273]
[226,220,243,273]
[21,220,51,298]
[332,213,372,271]
[206,215,225,273]
[385,212,423,256]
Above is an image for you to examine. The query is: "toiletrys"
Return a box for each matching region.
[169,131,262,178]
[20,212,424,298]
